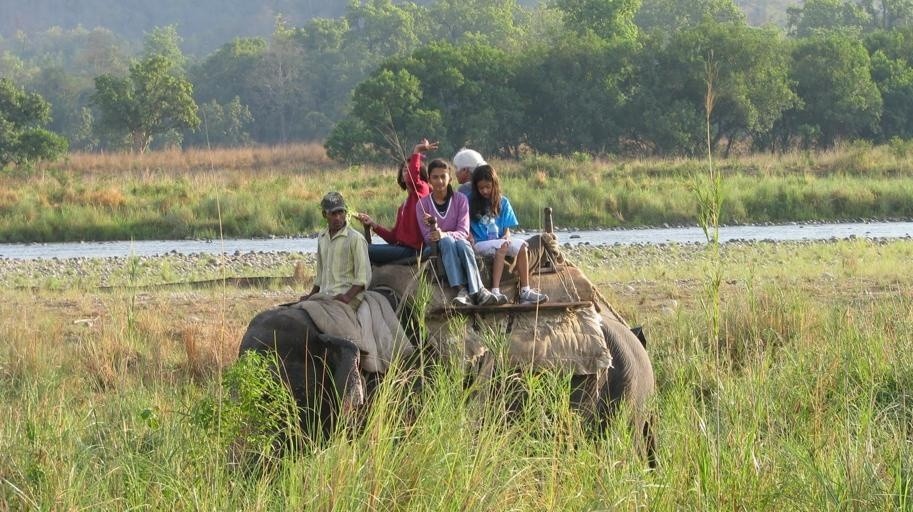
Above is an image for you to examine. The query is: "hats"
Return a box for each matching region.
[321,192,348,214]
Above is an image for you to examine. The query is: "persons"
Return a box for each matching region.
[453,147,487,199]
[414,159,497,307]
[298,190,373,312]
[355,139,440,264]
[467,165,549,305]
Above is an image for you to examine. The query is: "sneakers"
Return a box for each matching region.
[452,290,475,306]
[477,289,499,305]
[492,292,507,306]
[519,289,549,304]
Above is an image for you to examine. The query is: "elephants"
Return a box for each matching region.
[223,261,657,499]
